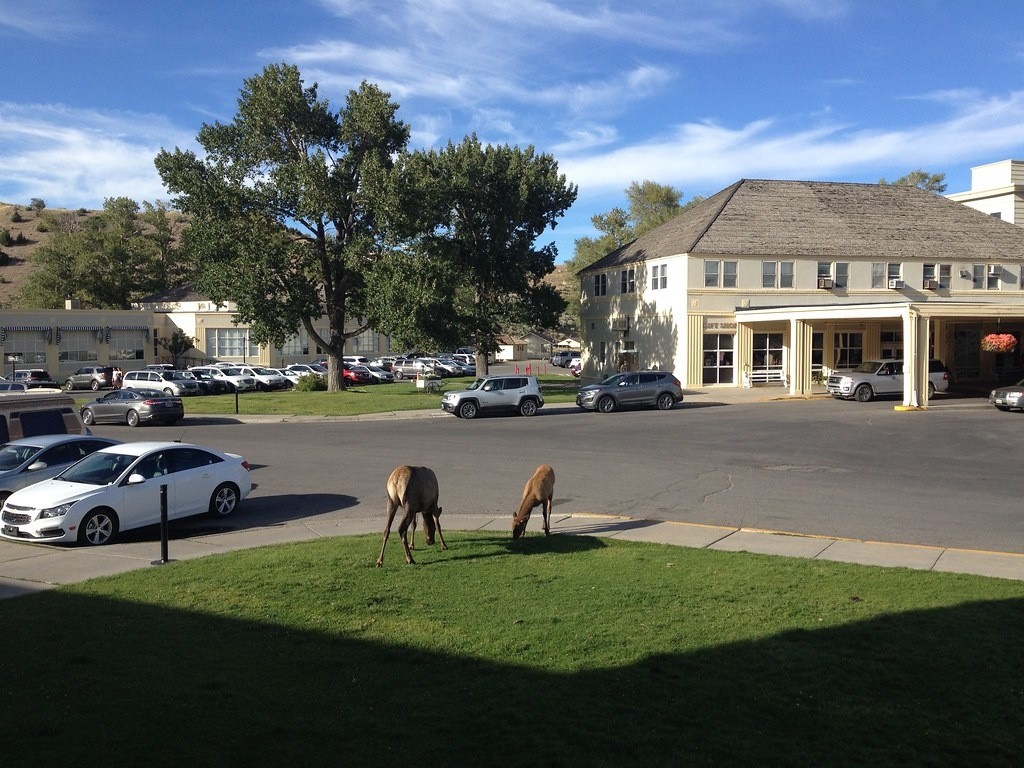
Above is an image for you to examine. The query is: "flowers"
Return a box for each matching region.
[981,333,1017,353]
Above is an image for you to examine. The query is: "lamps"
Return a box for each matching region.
[742,299,750,308]
[959,270,967,277]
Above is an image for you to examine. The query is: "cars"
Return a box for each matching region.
[64,364,123,391]
[988,378,1024,412]
[120,369,187,398]
[548,350,581,377]
[179,345,496,396]
[0,433,140,512]
[0,440,252,547]
[79,387,185,428]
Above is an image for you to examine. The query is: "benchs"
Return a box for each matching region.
[743,370,787,388]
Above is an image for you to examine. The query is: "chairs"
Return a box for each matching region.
[154,459,164,478]
[495,381,501,389]
[132,463,144,475]
[111,457,125,477]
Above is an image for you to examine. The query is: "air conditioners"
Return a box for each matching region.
[612,318,628,331]
[924,280,939,289]
[988,265,1003,275]
[889,280,905,288]
[819,280,834,288]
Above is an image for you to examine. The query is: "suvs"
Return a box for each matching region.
[0,368,93,447]
[440,373,546,420]
[575,369,684,413]
[826,356,950,403]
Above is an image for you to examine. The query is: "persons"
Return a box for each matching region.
[112,366,122,387]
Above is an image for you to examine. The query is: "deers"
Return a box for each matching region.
[375,464,448,569]
[511,463,556,543]
